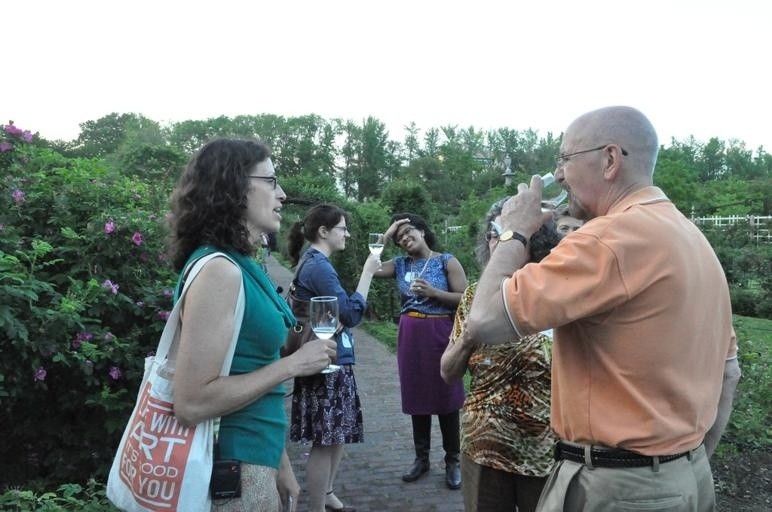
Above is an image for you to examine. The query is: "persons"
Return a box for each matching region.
[165,139,339,511]
[553,203,588,240]
[284,202,384,512]
[438,196,556,512]
[370,211,469,489]
[465,105,742,512]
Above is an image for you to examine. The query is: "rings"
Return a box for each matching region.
[417,286,422,291]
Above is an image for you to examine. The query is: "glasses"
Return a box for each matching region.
[247,175,277,189]
[335,226,347,232]
[395,227,416,244]
[553,144,628,170]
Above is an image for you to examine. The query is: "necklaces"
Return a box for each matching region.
[410,251,433,292]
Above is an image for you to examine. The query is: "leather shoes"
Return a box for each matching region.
[326,490,356,512]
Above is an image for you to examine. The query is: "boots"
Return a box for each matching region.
[403,432,430,482]
[443,438,462,489]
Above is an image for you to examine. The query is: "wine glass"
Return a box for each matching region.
[491,172,569,234]
[310,296,340,373]
[409,272,423,305]
[368,233,384,272]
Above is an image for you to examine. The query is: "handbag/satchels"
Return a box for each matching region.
[280,287,314,356]
[105,355,220,512]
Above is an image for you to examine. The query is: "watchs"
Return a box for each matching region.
[497,231,531,248]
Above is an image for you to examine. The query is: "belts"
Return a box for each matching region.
[552,440,689,468]
[408,311,446,318]
